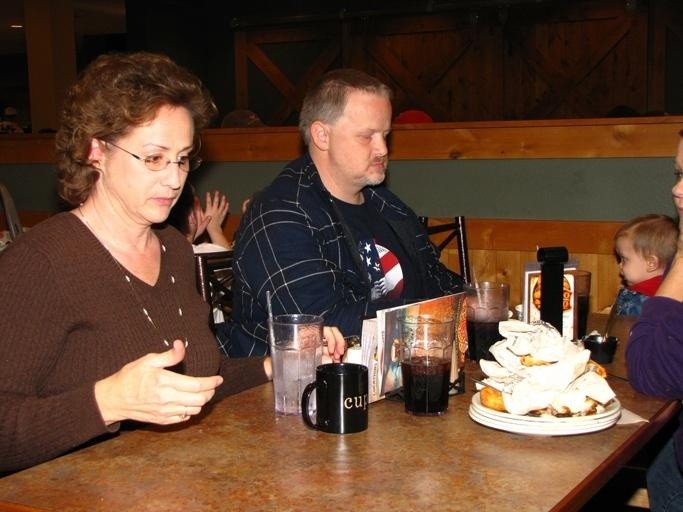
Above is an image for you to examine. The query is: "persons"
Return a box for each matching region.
[625,127,682,511]
[0,50,348,477]
[164,179,231,322]
[230,67,480,356]
[611,213,679,315]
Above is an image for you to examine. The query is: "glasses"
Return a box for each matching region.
[97,137,202,172]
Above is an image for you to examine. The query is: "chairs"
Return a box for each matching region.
[419,213,471,287]
[195,249,238,334]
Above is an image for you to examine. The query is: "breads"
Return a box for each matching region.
[474,320,616,416]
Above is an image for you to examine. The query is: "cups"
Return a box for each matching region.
[462,281,508,367]
[264,310,369,434]
[398,316,456,416]
[572,270,593,340]
[584,335,618,365]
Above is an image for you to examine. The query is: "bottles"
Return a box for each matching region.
[465,392,624,435]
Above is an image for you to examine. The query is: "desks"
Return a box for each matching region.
[0,304,677,512]
